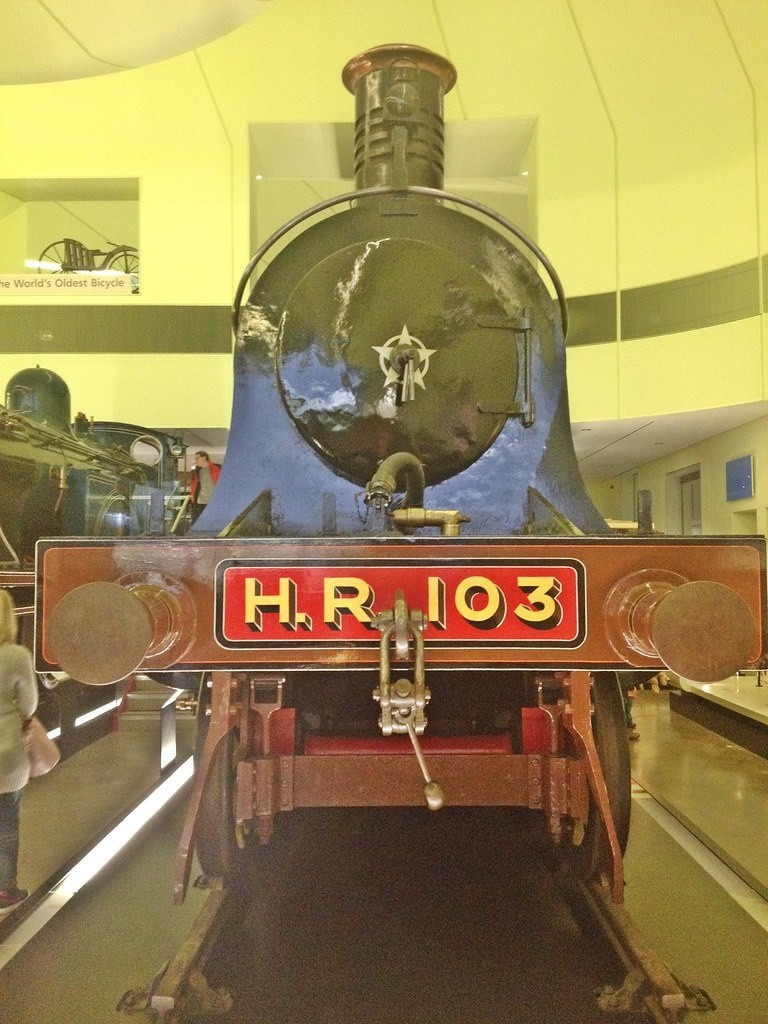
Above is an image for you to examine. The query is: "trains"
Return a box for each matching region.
[1,363,207,757]
[27,38,767,1024]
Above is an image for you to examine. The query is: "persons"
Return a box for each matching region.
[188,450,221,525]
[0,589,39,915]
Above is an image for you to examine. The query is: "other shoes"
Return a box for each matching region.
[0,888,28,908]
[629,730,640,739]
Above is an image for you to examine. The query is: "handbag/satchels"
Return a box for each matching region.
[23,716,61,778]
